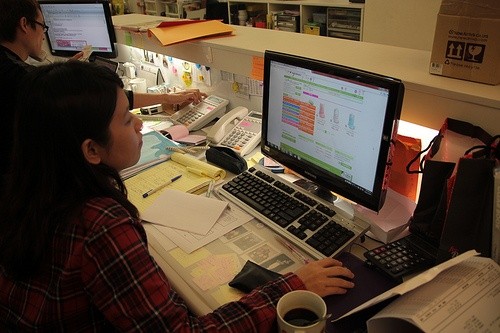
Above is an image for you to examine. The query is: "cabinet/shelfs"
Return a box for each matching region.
[145,0,202,19]
[226,0,367,42]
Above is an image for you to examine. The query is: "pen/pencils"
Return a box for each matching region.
[142,174,183,198]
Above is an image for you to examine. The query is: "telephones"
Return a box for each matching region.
[30,48,47,62]
[207,105,262,157]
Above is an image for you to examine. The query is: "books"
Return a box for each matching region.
[168,124,206,144]
[118,130,177,179]
[123,154,226,215]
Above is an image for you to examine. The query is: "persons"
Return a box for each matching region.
[0,60,355,333]
[0,0,208,110]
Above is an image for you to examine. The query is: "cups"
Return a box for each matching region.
[128,78,147,94]
[276,290,327,333]
[125,66,136,79]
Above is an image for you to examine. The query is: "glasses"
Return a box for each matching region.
[26,17,48,33]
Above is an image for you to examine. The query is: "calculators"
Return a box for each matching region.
[363,233,435,277]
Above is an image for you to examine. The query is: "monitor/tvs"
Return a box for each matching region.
[37,0,118,59]
[260,50,404,216]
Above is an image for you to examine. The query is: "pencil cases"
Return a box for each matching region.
[205,144,248,175]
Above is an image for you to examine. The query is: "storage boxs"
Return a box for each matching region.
[271,12,299,32]
[429,0,500,85]
[303,8,362,40]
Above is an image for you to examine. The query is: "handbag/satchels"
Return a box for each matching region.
[404,117,499,265]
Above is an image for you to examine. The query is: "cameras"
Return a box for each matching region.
[265,166,284,173]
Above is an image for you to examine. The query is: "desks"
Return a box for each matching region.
[119,112,422,316]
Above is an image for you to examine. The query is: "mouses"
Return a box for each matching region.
[333,251,362,281]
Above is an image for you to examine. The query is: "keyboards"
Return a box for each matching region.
[218,162,371,260]
